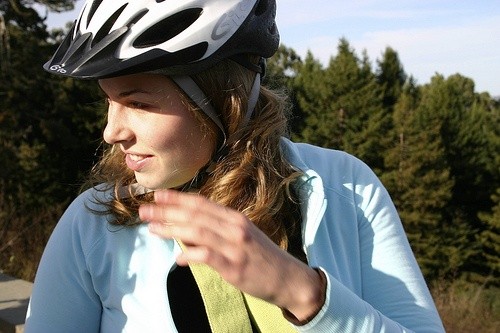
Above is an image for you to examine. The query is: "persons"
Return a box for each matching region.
[19,0,451,333]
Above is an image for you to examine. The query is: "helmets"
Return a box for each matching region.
[42,0,280,80]
